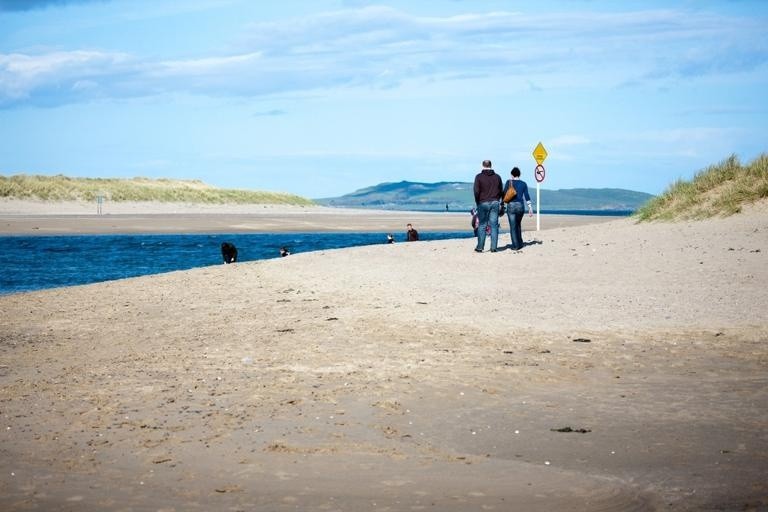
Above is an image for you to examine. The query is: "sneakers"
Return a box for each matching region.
[499,207,506,217]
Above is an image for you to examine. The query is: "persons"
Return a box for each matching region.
[220,241,238,265]
[280,247,290,258]
[470,208,500,237]
[501,167,534,250]
[406,223,418,241]
[386,233,396,243]
[473,160,503,252]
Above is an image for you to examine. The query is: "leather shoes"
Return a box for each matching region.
[474,248,482,252]
[491,249,497,252]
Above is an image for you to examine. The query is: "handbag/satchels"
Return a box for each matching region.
[503,188,517,203]
